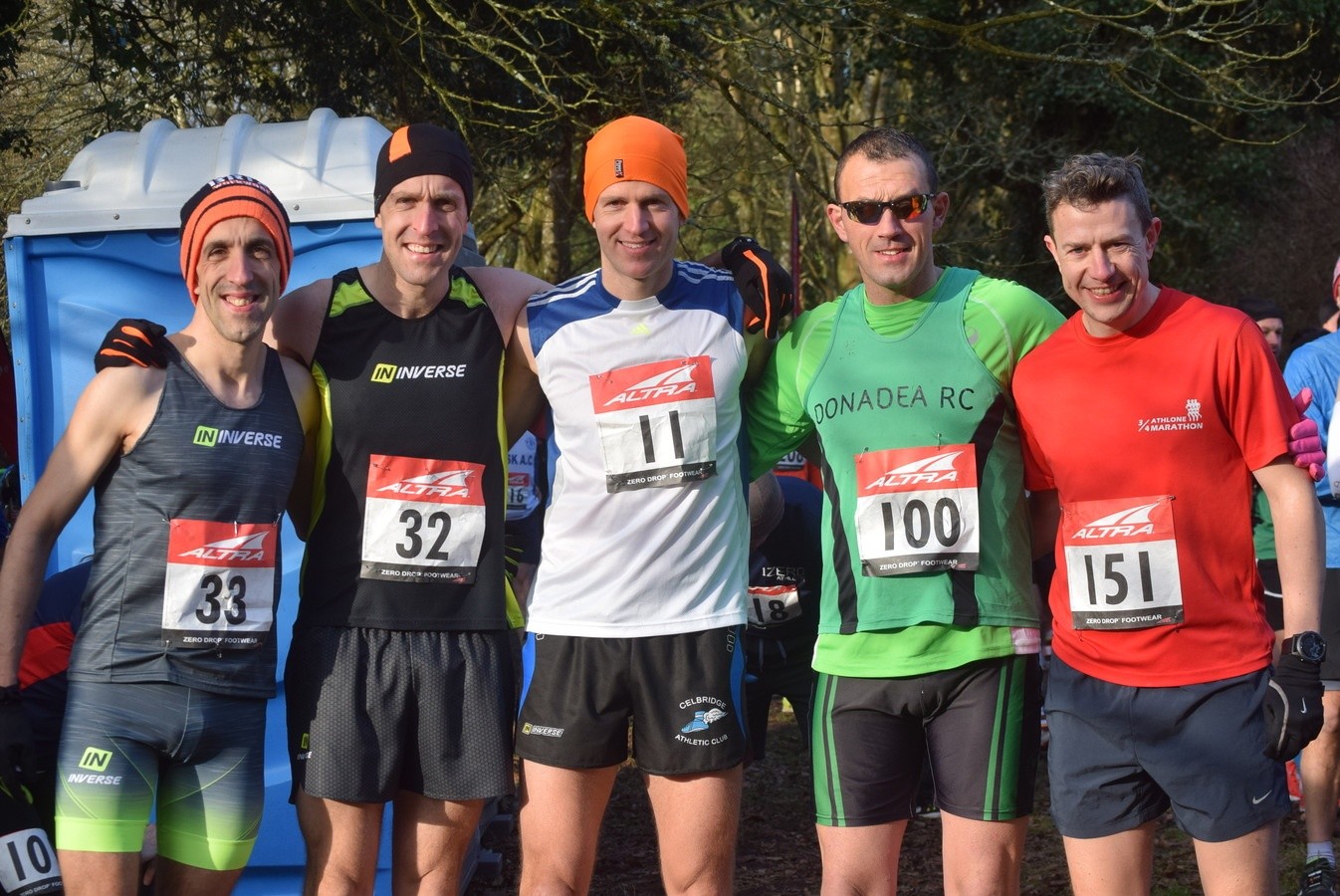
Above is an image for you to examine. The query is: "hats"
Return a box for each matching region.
[375,125,475,218]
[179,175,293,306]
[583,116,689,223]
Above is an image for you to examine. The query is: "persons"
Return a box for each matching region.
[1010,154,1323,896]
[499,115,818,896]
[0,258,1340,896]
[95,127,795,896]
[515,131,1326,896]
[0,175,517,896]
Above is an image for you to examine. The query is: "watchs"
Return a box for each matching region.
[1282,632,1327,664]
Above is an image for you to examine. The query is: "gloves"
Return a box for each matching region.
[1287,387,1325,481]
[1263,635,1324,760]
[94,317,167,373]
[720,236,795,341]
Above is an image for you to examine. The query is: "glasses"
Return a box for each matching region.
[838,193,938,225]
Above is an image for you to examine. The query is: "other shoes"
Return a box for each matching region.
[1299,856,1335,896]
